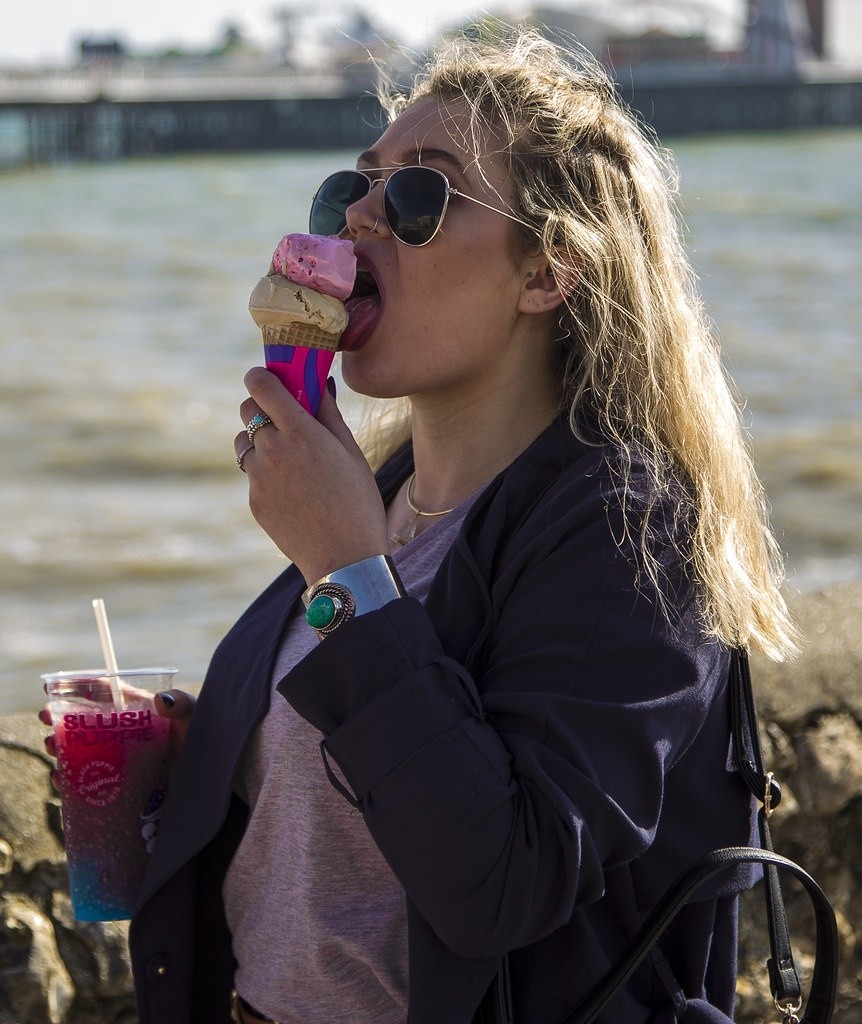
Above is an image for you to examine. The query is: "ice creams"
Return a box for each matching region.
[248,231,356,419]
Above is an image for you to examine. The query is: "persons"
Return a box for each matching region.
[39,36,797,1024]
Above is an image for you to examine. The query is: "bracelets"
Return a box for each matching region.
[140,808,166,825]
[302,556,402,643]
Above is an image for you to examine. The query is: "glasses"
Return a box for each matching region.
[309,166,562,249]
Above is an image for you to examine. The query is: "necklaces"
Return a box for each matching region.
[391,472,454,546]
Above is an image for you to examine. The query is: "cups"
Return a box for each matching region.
[44,667,193,923]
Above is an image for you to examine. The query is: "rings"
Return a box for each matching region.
[236,444,255,473]
[247,414,271,443]
